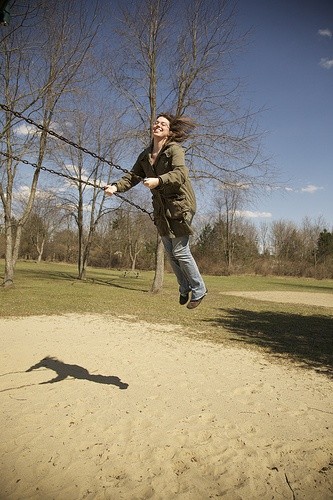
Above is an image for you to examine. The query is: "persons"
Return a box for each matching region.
[104,112,208,309]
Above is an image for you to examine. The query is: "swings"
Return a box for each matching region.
[0,103,197,237]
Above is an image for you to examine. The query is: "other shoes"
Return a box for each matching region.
[180,287,192,304]
[187,288,208,308]
